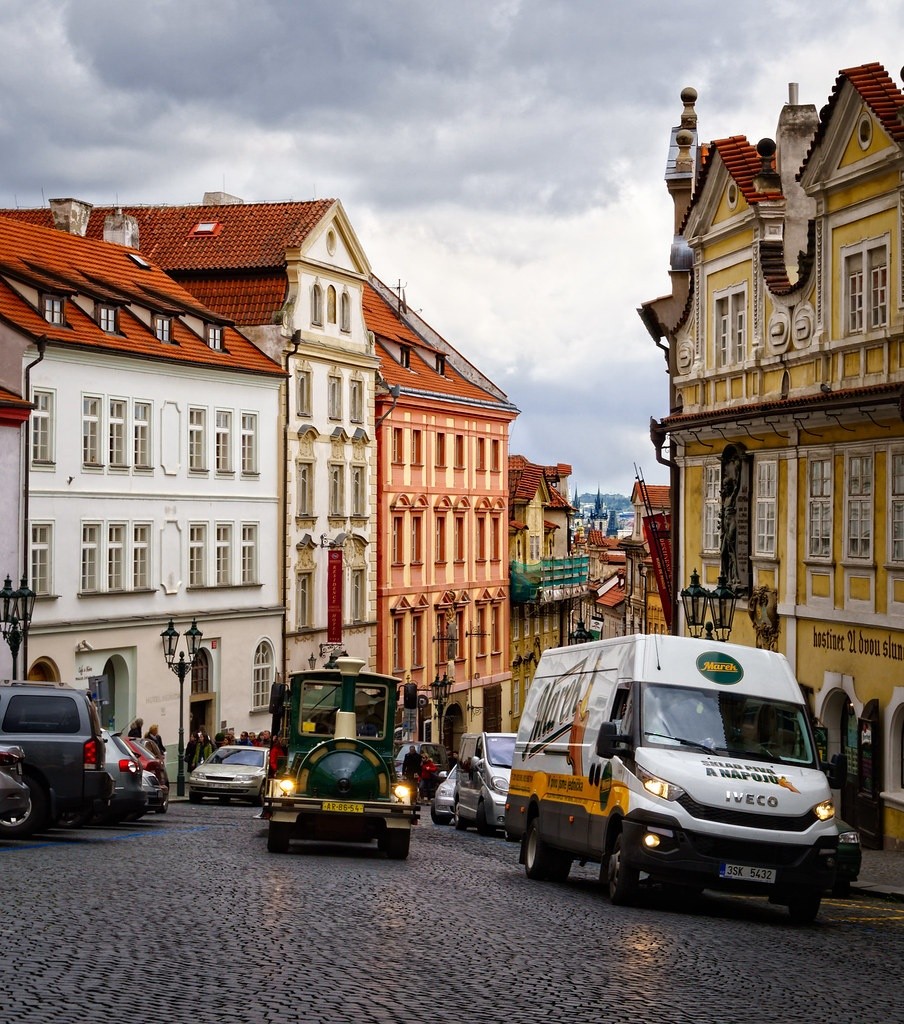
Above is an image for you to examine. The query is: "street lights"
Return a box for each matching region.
[0,572,36,684]
[430,671,456,743]
[159,616,204,797]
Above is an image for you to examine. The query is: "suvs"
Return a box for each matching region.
[0,679,116,830]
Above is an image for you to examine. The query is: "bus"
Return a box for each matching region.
[262,657,422,860]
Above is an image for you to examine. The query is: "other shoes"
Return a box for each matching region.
[425,802,431,806]
[253,813,268,819]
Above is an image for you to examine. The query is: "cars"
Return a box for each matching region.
[395,740,449,799]
[430,763,458,825]
[832,818,862,897]
[122,735,171,814]
[95,729,148,826]
[189,744,269,806]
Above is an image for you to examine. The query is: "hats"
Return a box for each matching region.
[227,730,234,736]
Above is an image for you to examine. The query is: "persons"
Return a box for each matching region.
[127,717,286,818]
[401,746,465,807]
[731,721,783,757]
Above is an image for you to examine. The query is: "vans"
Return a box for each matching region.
[453,732,518,837]
[503,634,840,924]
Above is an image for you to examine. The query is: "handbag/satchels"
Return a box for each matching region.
[184,755,189,762]
[428,771,439,782]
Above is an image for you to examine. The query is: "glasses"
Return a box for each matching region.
[422,757,426,759]
[241,734,244,737]
